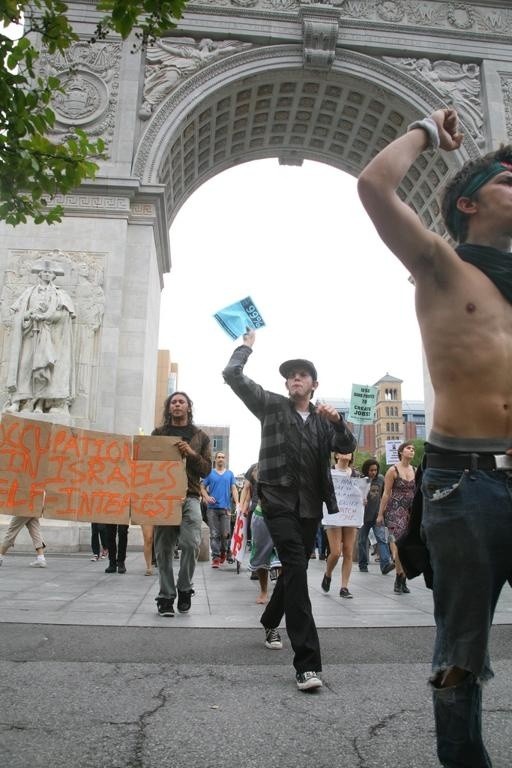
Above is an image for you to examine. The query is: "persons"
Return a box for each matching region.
[151,393,212,616]
[91,523,181,576]
[353,105,512,768]
[199,452,282,604]
[224,327,356,693]
[0,517,50,568]
[311,442,418,598]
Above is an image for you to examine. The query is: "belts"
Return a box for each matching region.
[420,451,508,474]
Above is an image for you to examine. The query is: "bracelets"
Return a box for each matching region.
[408,118,441,151]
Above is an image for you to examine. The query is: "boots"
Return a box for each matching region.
[118,560,125,573]
[402,577,410,592]
[394,573,403,594]
[104,559,117,573]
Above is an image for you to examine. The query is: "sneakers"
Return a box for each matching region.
[360,568,368,572]
[156,598,175,616]
[29,559,46,568]
[212,560,220,567]
[382,561,395,573]
[220,552,226,563]
[321,574,332,591]
[261,620,283,650]
[292,668,322,691]
[340,588,353,598]
[177,589,194,611]
[91,551,109,561]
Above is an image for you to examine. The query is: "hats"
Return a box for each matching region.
[279,356,320,380]
